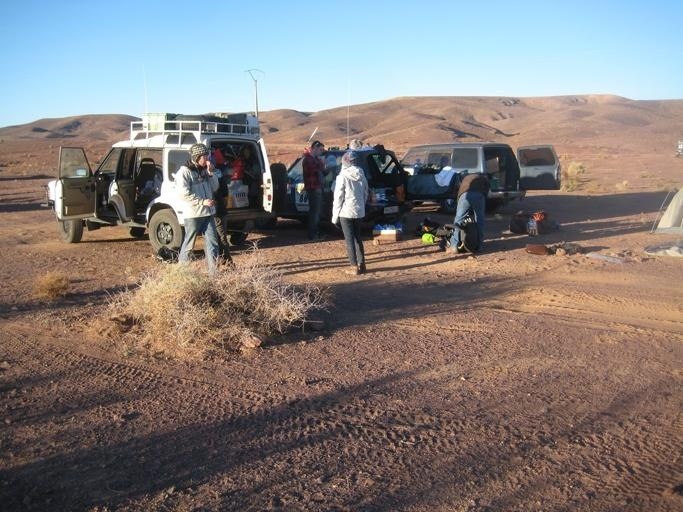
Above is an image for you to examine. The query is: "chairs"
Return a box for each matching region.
[134,154,157,187]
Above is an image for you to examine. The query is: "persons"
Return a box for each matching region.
[445,174,491,254]
[331,153,369,275]
[302,140,330,239]
[210,169,233,267]
[174,144,221,272]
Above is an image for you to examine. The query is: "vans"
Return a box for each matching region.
[392,144,562,218]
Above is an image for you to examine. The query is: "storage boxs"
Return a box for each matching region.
[373,227,401,242]
[509,209,546,236]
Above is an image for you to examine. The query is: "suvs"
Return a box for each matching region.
[44,112,287,259]
[278,144,407,234]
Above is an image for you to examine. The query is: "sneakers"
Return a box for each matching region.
[345,265,359,274]
[358,263,366,272]
[446,246,459,253]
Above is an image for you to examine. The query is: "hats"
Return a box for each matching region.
[341,151,360,168]
[189,144,209,166]
